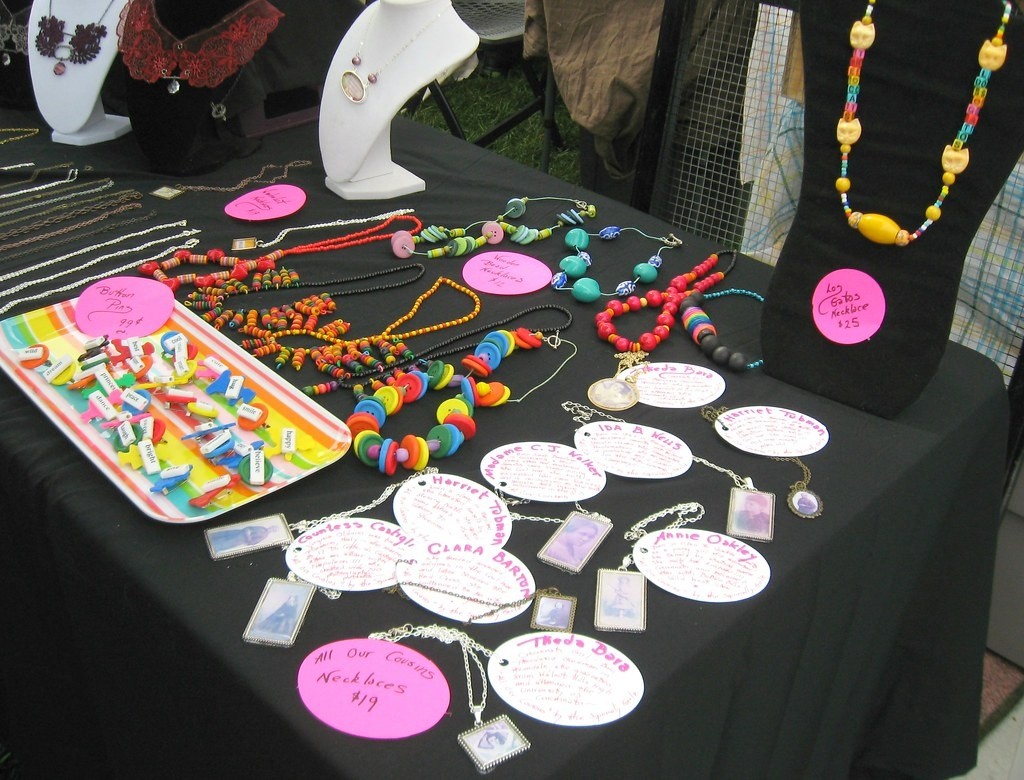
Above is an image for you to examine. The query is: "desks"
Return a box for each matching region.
[1,97,1010,780]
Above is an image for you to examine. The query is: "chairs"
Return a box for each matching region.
[405,0,564,154]
